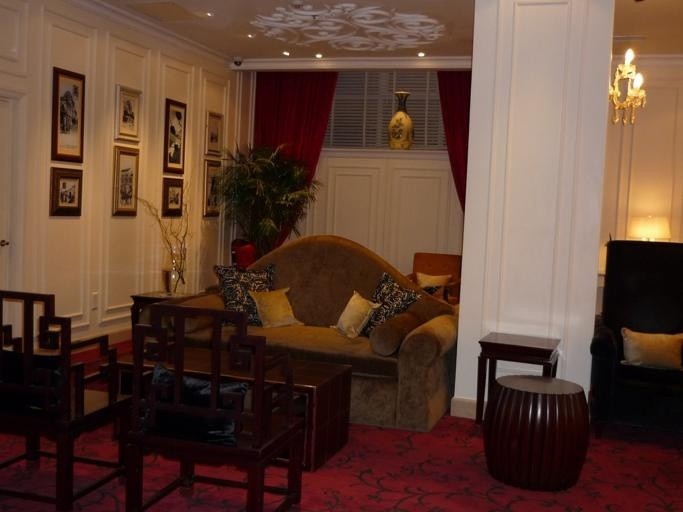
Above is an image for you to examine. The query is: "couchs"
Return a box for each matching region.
[164,236,459,432]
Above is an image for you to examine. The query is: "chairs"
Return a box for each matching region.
[413,253,462,306]
[590,241,683,433]
[0,290,131,512]
[125,303,305,512]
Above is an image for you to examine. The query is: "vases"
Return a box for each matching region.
[167,269,179,294]
[388,91,414,150]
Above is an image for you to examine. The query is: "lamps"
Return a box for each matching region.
[627,214,671,242]
[609,48,647,126]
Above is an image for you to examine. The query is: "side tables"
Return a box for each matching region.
[475,332,560,424]
[130,291,193,340]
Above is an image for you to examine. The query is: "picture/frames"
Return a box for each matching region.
[204,109,224,217]
[112,83,142,217]
[51,65,85,217]
[162,98,187,218]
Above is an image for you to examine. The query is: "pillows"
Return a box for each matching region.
[3,349,63,419]
[329,272,421,356]
[213,263,305,329]
[416,272,452,296]
[143,362,248,465]
[620,326,683,371]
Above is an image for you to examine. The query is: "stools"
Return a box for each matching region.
[483,375,590,491]
